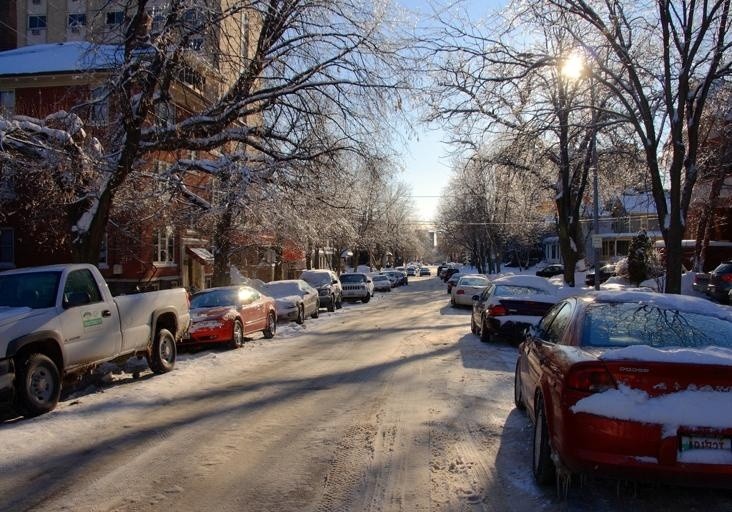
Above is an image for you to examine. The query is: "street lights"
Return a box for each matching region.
[560,55,599,293]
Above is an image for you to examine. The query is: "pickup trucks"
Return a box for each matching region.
[0,264,190,418]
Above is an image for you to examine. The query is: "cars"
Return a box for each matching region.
[260,280,320,323]
[536,264,564,278]
[471,279,559,343]
[585,265,616,286]
[437,265,492,307]
[692,264,732,303]
[514,295,731,483]
[420,268,431,277]
[339,264,417,303]
[177,285,278,348]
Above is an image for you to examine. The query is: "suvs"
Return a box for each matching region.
[298,269,343,312]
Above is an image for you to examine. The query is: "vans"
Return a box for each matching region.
[654,239,731,271]
